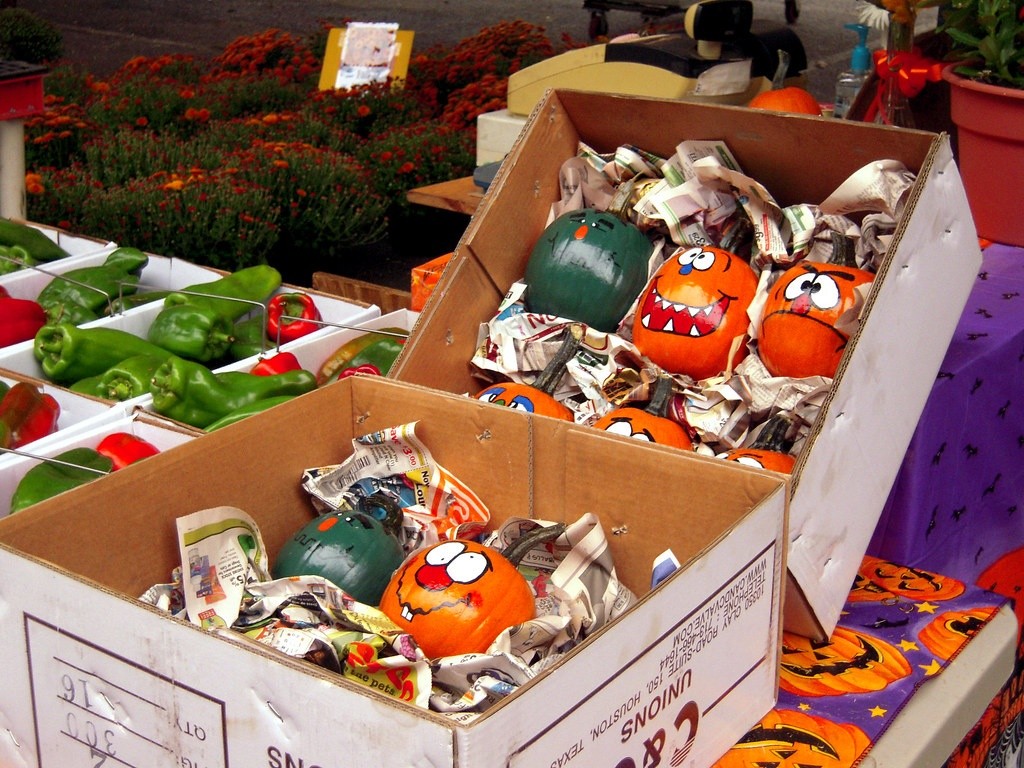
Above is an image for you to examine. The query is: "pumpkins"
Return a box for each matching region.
[746,48,823,116]
[269,493,568,659]
[469,176,878,476]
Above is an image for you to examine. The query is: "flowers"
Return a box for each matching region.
[24,17,683,266]
[858,0,936,25]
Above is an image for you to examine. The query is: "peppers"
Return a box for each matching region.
[1,213,411,514]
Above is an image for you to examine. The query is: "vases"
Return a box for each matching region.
[864,15,915,129]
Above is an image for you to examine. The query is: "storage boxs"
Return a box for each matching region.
[0,374,794,768]
[0,217,119,282]
[0,248,224,355]
[134,308,420,434]
[384,88,983,643]
[0,277,381,412]
[0,369,125,465]
[0,413,205,521]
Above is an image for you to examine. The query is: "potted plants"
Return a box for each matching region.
[937,0,1024,247]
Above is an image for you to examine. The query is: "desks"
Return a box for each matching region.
[685,549,1017,768]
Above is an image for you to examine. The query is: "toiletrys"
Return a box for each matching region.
[832,23,877,120]
